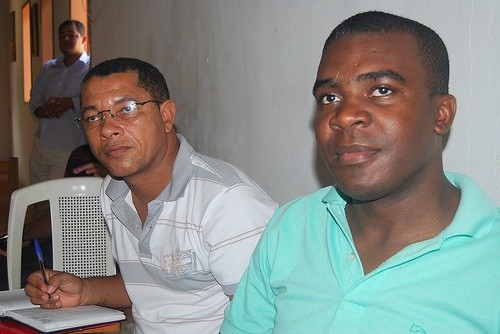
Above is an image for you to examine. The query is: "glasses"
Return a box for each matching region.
[74,99,164,131]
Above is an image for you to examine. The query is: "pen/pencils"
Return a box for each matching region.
[33,239,53,310]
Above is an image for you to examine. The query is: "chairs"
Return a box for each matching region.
[0,177,116,290]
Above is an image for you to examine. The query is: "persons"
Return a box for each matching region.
[29,20,90,217]
[1,58,279,334]
[218,10,500,334]
